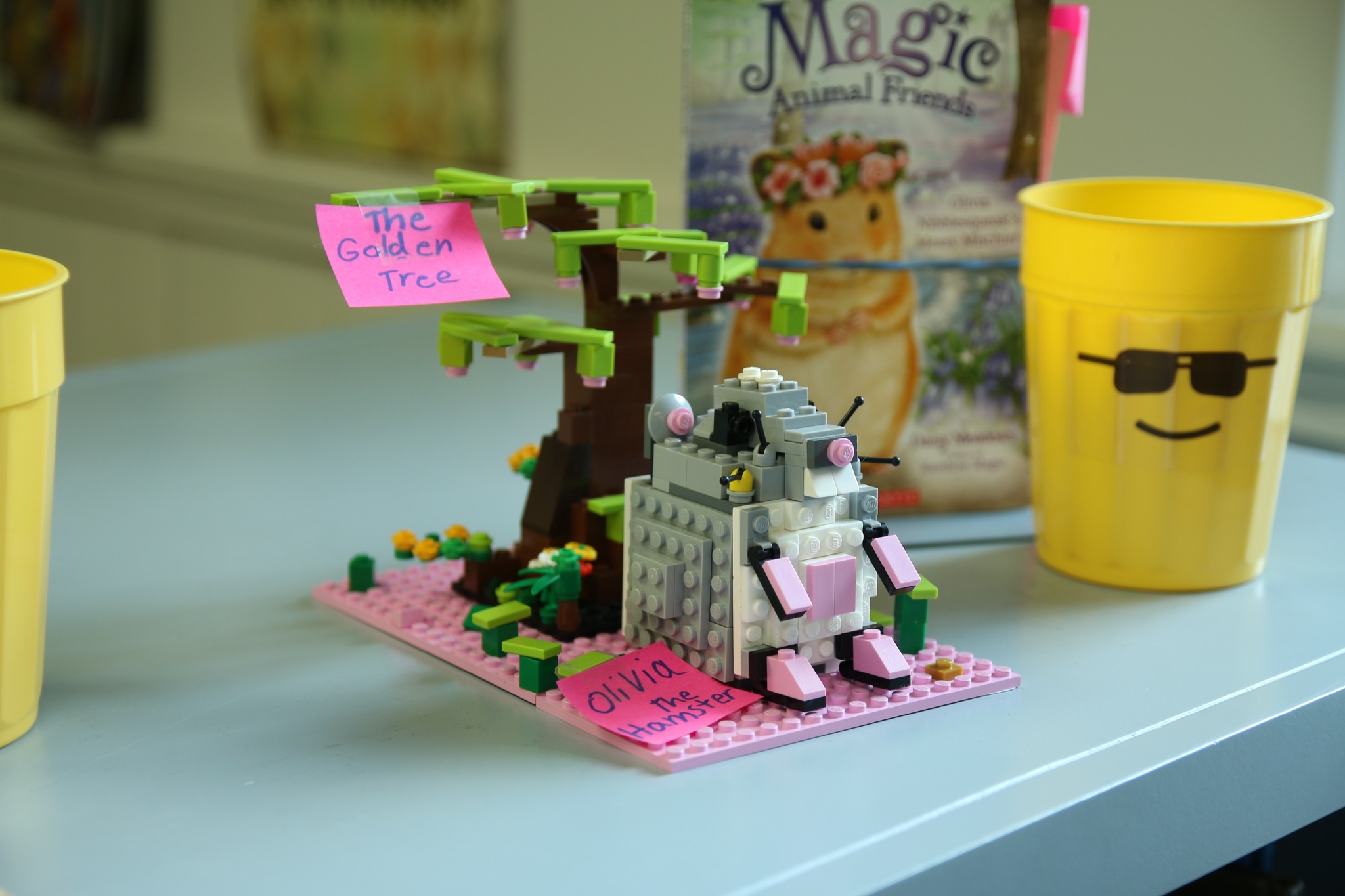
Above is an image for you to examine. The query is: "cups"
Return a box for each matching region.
[1015,176,1335,591]
[0,247,70,749]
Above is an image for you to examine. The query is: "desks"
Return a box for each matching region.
[0,312,1344,895]
